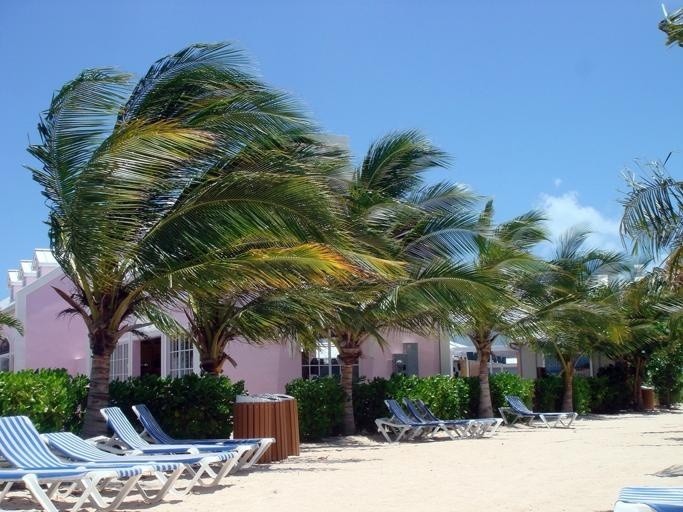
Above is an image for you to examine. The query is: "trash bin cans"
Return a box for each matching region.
[232,393,300,464]
[641,388,654,411]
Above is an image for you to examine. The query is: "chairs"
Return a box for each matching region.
[374,397,503,446]
[0,403,276,512]
[614,486,683,512]
[498,396,578,429]
